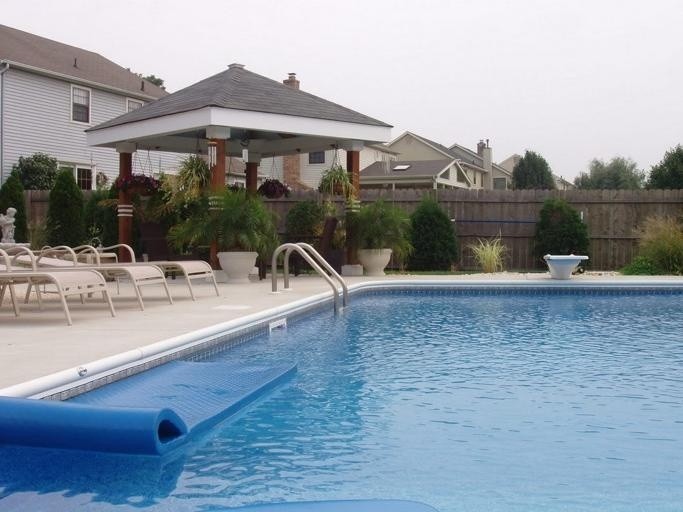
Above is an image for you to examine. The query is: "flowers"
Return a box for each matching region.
[114,172,161,192]
[257,179,290,196]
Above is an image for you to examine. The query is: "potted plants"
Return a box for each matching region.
[165,181,283,284]
[318,164,360,202]
[160,153,214,208]
[335,189,417,276]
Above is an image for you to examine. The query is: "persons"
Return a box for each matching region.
[0,207,17,240]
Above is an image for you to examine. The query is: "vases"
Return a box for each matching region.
[263,191,284,199]
[122,183,157,196]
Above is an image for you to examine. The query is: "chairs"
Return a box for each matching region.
[0,243,221,327]
